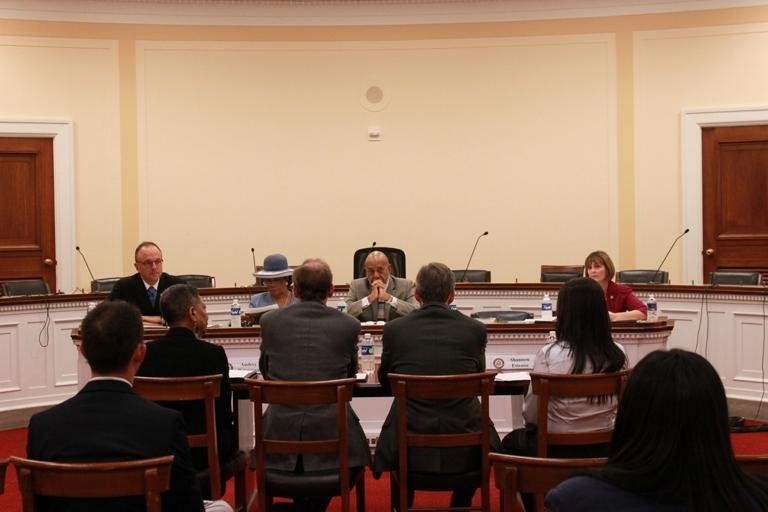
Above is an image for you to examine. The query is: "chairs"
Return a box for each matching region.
[471,311,531,321]
[453,270,492,282]
[9,455,174,511]
[488,452,605,512]
[354,247,406,280]
[245,373,367,511]
[615,270,669,283]
[529,368,633,459]
[709,272,761,284]
[541,265,584,282]
[387,370,497,512]
[0,279,51,297]
[90,277,121,292]
[176,275,216,287]
[133,377,248,511]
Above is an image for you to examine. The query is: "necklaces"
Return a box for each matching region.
[273,291,292,307]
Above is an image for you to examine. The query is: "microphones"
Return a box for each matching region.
[370,241,376,252]
[251,248,257,272]
[648,229,689,283]
[76,246,99,294]
[461,231,488,281]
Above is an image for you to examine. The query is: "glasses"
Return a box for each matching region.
[136,259,163,269]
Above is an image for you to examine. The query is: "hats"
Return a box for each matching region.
[253,255,294,279]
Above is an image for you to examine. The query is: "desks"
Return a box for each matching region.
[231,370,532,472]
[0,284,768,412]
[70,315,675,454]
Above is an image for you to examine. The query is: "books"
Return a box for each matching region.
[228,370,258,384]
[240,303,280,327]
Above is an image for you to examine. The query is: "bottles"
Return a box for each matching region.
[541,295,552,321]
[230,300,241,328]
[361,333,375,375]
[546,331,557,346]
[647,294,658,322]
[449,300,456,309]
[336,298,348,313]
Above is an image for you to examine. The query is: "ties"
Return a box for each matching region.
[148,287,157,310]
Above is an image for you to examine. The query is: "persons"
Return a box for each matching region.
[108,241,182,327]
[584,250,647,322]
[133,283,235,502]
[241,253,299,325]
[26,300,206,512]
[248,258,370,512]
[500,276,633,512]
[372,262,501,511]
[545,348,768,512]
[345,251,421,322]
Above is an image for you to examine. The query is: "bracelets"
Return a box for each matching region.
[384,295,394,304]
